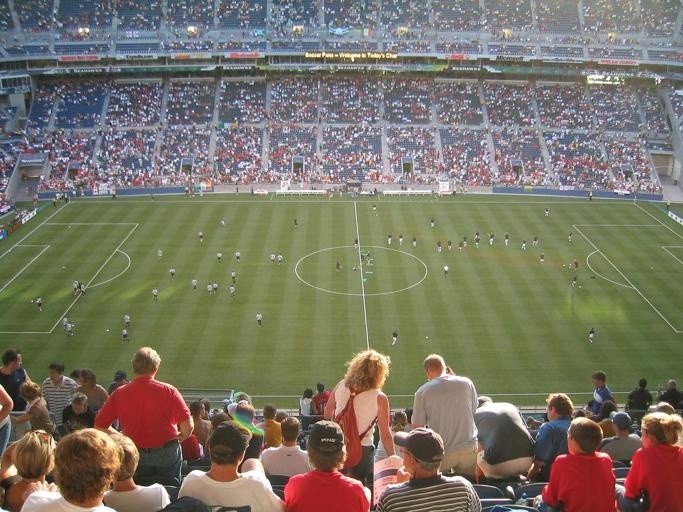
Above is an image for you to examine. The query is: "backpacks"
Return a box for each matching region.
[334,386,377,469]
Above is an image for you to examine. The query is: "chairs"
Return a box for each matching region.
[610,460,629,479]
[472,484,505,497]
[181,459,212,474]
[268,474,291,490]
[134,475,181,503]
[516,482,549,498]
[484,505,540,512]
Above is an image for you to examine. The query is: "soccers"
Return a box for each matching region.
[579,286,581,288]
[71,333,73,336]
[563,264,565,267]
[30,300,33,303]
[106,329,109,332]
[569,266,571,269]
[68,226,71,229]
[44,216,47,220]
[61,266,66,270]
[8,249,11,253]
[650,267,653,270]
[72,324,74,326]
[425,336,429,340]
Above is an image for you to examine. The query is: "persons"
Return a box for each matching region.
[2,0,683,345]
[1,346,683,511]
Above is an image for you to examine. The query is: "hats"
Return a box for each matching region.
[610,411,631,426]
[309,421,344,453]
[209,421,252,455]
[393,428,445,464]
